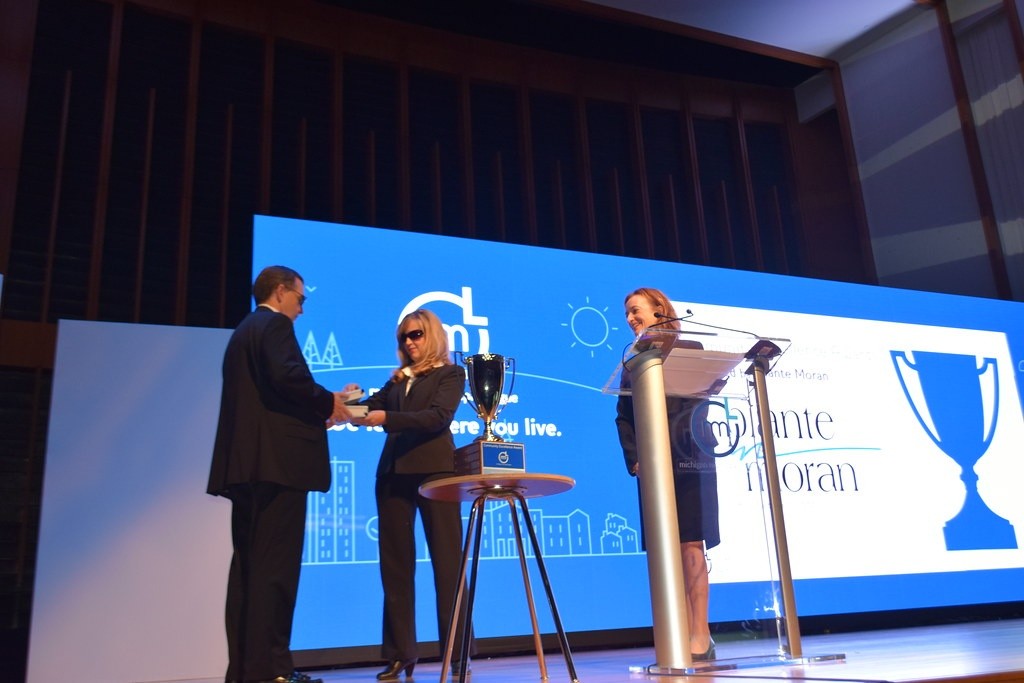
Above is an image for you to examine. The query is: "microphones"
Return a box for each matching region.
[635,309,782,359]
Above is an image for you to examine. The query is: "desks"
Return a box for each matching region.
[417,473,577,683]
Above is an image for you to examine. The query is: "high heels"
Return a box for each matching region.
[691,636,716,660]
[451,656,472,676]
[377,656,418,680]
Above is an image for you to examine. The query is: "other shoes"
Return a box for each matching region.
[249,675,323,683]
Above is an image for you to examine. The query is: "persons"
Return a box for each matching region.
[206,266,355,683]
[615,290,722,663]
[344,309,470,679]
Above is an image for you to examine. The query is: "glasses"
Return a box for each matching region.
[401,329,424,343]
[289,289,305,305]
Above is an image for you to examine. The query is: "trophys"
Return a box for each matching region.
[454,351,515,444]
